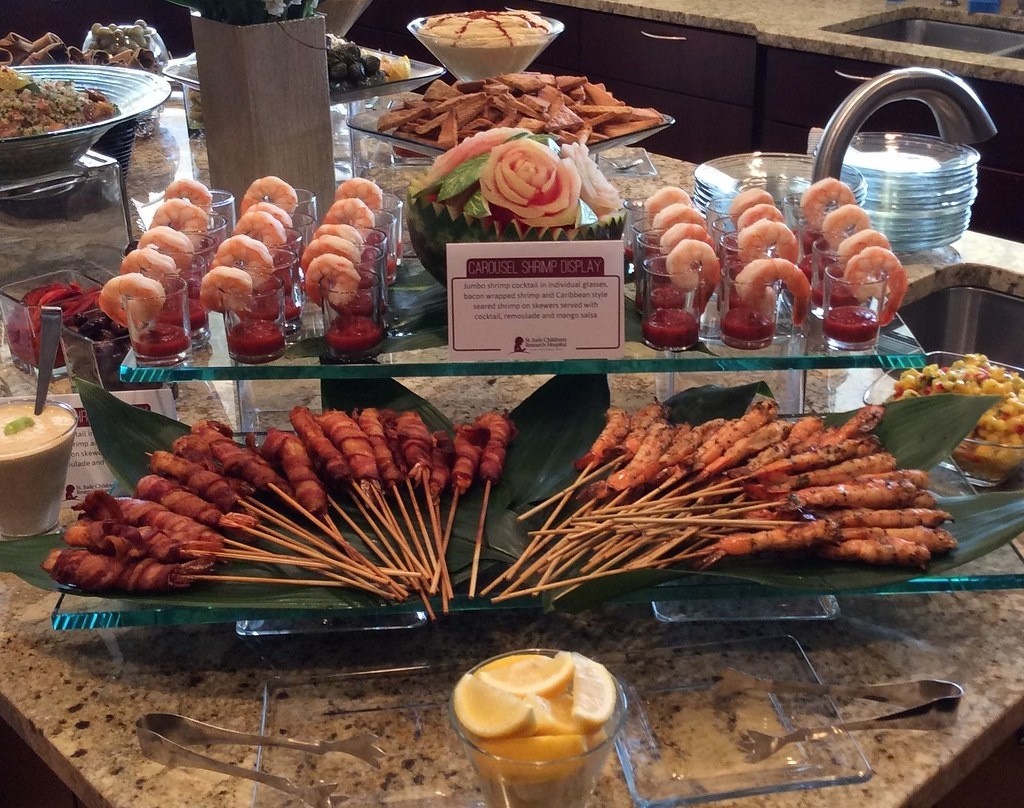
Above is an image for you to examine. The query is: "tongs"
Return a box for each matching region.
[136,712,386,808]
[696,666,964,763]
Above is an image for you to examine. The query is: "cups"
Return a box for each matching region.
[449,648,627,808]
[0,398,78,539]
[409,14,565,82]
[123,189,403,368]
[60,314,177,399]
[624,197,889,351]
[0,269,103,382]
[82,26,168,74]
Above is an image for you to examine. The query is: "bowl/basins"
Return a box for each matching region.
[865,351,1024,488]
[0,64,171,169]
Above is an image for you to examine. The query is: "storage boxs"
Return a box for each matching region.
[191,15,334,223]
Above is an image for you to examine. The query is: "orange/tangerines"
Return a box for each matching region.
[452,653,616,781]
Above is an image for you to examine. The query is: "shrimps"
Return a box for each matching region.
[573,394,959,567]
[642,173,911,327]
[96,174,384,328]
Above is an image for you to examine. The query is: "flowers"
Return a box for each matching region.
[169,0,322,25]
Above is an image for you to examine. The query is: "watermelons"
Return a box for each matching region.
[405,127,628,290]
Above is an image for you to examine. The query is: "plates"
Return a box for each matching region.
[162,48,447,105]
[346,104,675,158]
[591,635,869,808]
[593,148,658,178]
[253,658,486,808]
[693,131,981,252]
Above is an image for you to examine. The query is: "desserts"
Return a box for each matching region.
[415,9,550,83]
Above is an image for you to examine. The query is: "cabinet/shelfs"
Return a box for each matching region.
[50,262,1024,631]
[343,0,1024,244]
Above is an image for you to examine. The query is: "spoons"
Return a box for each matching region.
[598,156,642,170]
